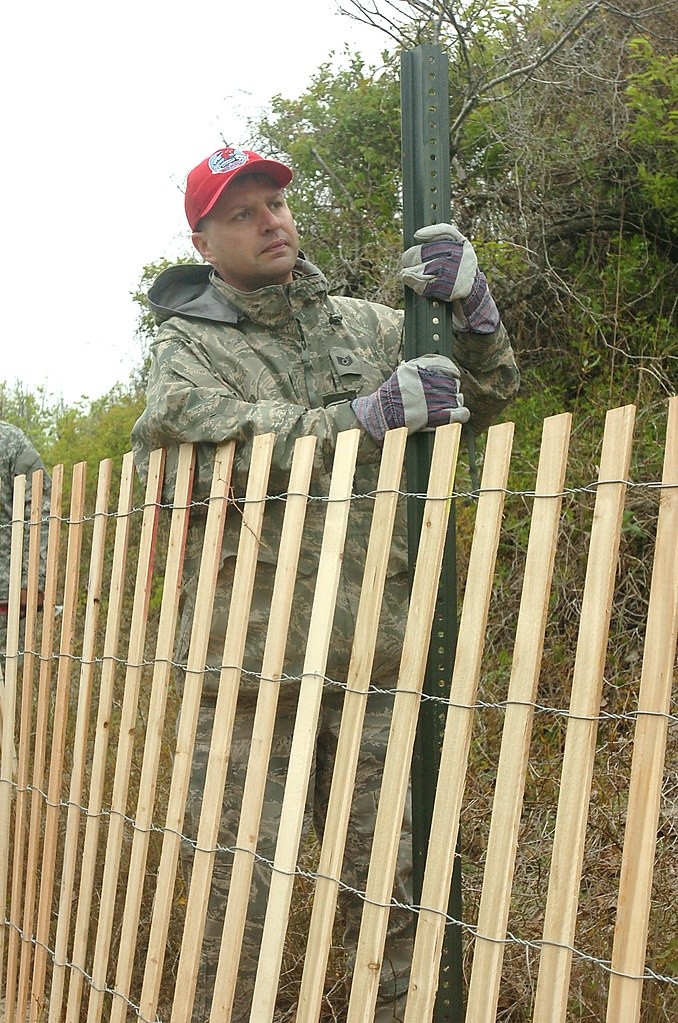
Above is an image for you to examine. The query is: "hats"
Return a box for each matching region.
[184,148,292,233]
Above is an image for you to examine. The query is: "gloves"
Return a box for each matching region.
[399,223,501,335]
[350,353,471,447]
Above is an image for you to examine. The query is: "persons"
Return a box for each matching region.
[132,147,518,1019]
[0,423,60,675]
